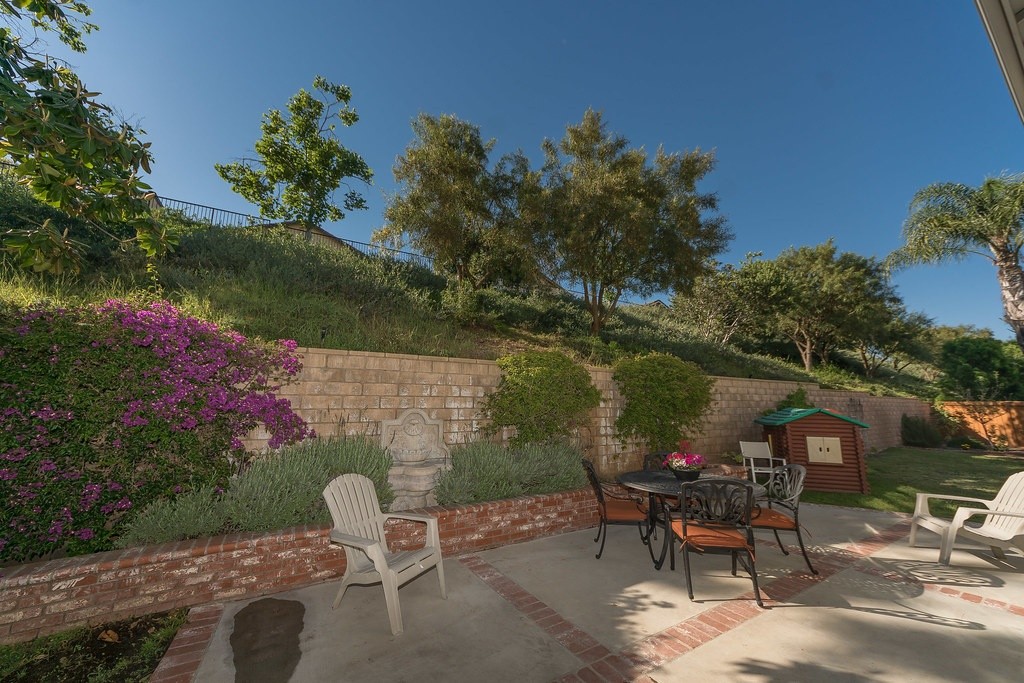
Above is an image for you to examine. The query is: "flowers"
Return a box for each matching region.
[660,447,709,472]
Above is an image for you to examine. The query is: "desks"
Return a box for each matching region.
[622,471,768,579]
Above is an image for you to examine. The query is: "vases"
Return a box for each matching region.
[669,468,701,486]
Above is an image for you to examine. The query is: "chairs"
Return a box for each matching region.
[909,471,1024,564]
[322,472,449,637]
[581,440,819,608]
